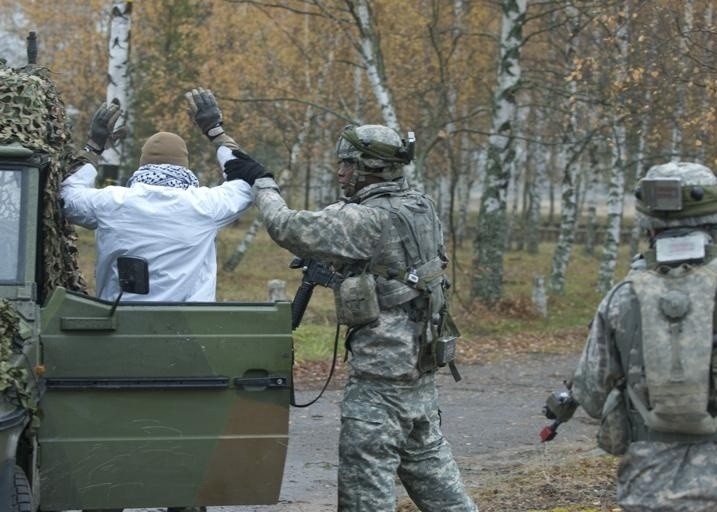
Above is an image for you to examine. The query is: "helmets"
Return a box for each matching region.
[635,162,717,235]
[334,124,407,181]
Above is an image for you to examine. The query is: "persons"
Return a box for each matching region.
[223,124,478,512]
[573,161,717,512]
[56,87,254,512]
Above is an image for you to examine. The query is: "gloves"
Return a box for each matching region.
[186,87,224,135]
[224,149,274,186]
[87,103,128,150]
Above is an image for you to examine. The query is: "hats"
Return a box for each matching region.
[141,131,189,167]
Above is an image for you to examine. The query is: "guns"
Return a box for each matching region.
[541,381,580,442]
[289,256,343,330]
